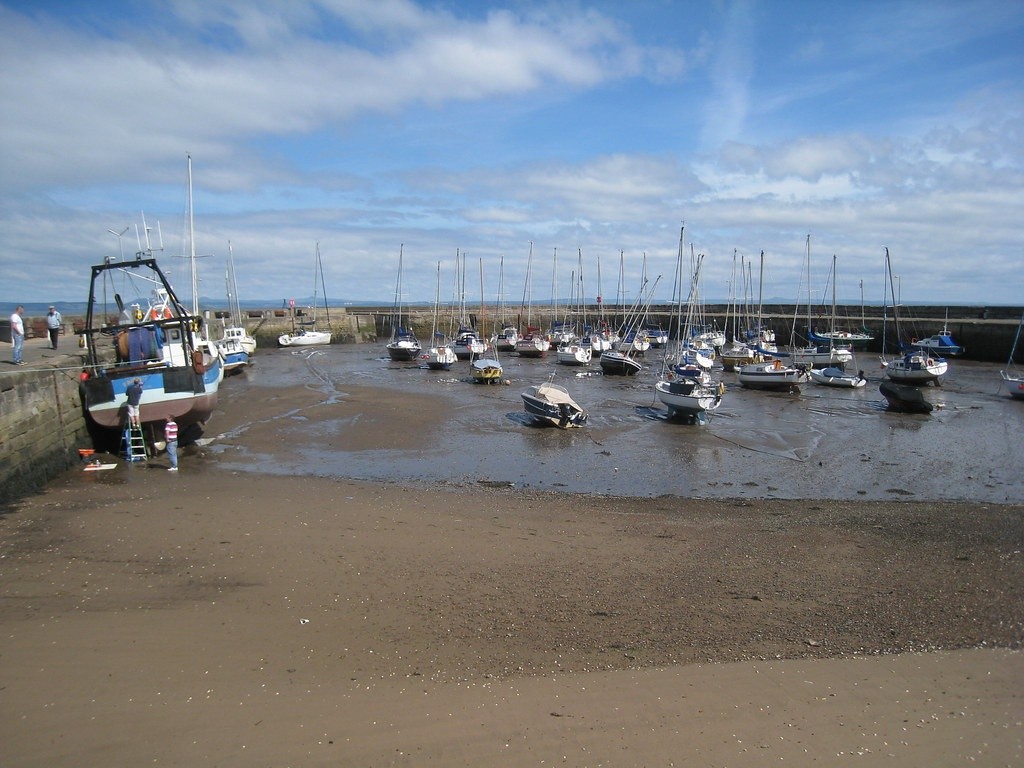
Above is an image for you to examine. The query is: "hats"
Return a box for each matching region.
[49,306,54,309]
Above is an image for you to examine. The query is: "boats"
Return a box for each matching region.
[733,358,814,388]
[910,306,960,358]
[80,251,224,430]
[520,369,588,429]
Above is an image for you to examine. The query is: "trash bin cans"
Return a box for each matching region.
[298,308,302,317]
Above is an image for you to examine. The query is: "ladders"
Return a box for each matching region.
[118,416,148,463]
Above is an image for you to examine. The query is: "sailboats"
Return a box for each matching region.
[654,227,726,425]
[545,248,576,348]
[785,234,854,371]
[806,254,876,352]
[446,247,489,358]
[384,243,422,361]
[277,241,333,347]
[466,258,504,384]
[424,260,460,370]
[809,254,868,388]
[492,255,524,353]
[999,315,1024,396]
[572,241,779,371]
[515,242,551,356]
[212,241,257,371]
[555,270,593,364]
[599,274,662,374]
[880,245,949,383]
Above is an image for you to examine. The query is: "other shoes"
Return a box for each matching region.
[132,424,139,429]
[17,359,28,366]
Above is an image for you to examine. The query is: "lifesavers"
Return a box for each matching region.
[152,305,171,320]
[467,345,471,349]
[195,316,203,332]
[709,353,715,360]
[720,382,724,395]
[926,358,935,366]
[765,344,769,348]
[426,355,430,359]
[290,333,293,337]
[646,338,650,342]
[519,334,523,341]
[290,299,294,308]
[421,355,425,359]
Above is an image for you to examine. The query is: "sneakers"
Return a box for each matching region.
[168,466,178,471]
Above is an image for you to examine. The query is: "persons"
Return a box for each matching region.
[11,305,28,365]
[296,326,305,336]
[47,306,61,350]
[164,414,178,471]
[126,379,143,429]
[112,337,121,366]
